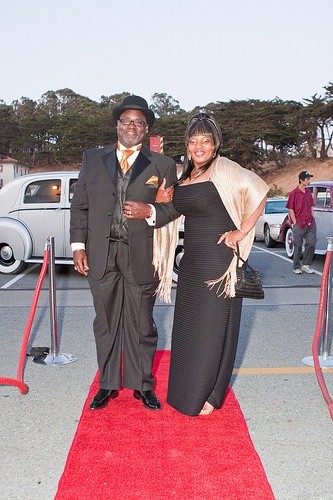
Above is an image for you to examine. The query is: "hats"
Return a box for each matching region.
[112,96,155,130]
[298,171,313,178]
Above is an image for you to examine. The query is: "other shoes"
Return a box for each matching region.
[293,268,302,274]
[301,264,314,274]
[199,401,214,416]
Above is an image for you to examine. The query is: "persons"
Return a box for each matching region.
[156,113,270,417]
[285,170,317,274]
[70,95,181,410]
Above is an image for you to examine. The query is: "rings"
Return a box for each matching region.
[129,210,131,215]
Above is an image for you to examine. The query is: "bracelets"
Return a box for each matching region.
[241,229,247,235]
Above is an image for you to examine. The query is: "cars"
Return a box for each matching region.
[279,180,333,259]
[0,170,185,284]
[253,197,290,248]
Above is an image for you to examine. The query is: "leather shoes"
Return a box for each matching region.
[90,388,119,410]
[133,390,162,410]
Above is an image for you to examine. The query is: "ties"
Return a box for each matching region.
[120,150,135,174]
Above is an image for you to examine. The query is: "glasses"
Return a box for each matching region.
[118,119,147,127]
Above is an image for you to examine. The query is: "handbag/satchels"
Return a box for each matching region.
[234,242,265,300]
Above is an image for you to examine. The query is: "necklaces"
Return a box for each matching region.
[189,170,201,183]
